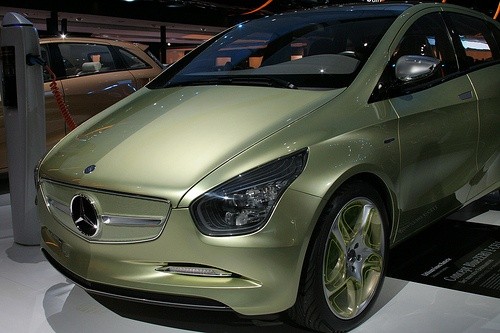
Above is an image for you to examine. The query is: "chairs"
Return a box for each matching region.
[78,61,102,75]
[353,23,427,66]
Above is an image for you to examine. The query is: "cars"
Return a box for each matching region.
[35,4,500,333]
[0,37,166,175]
